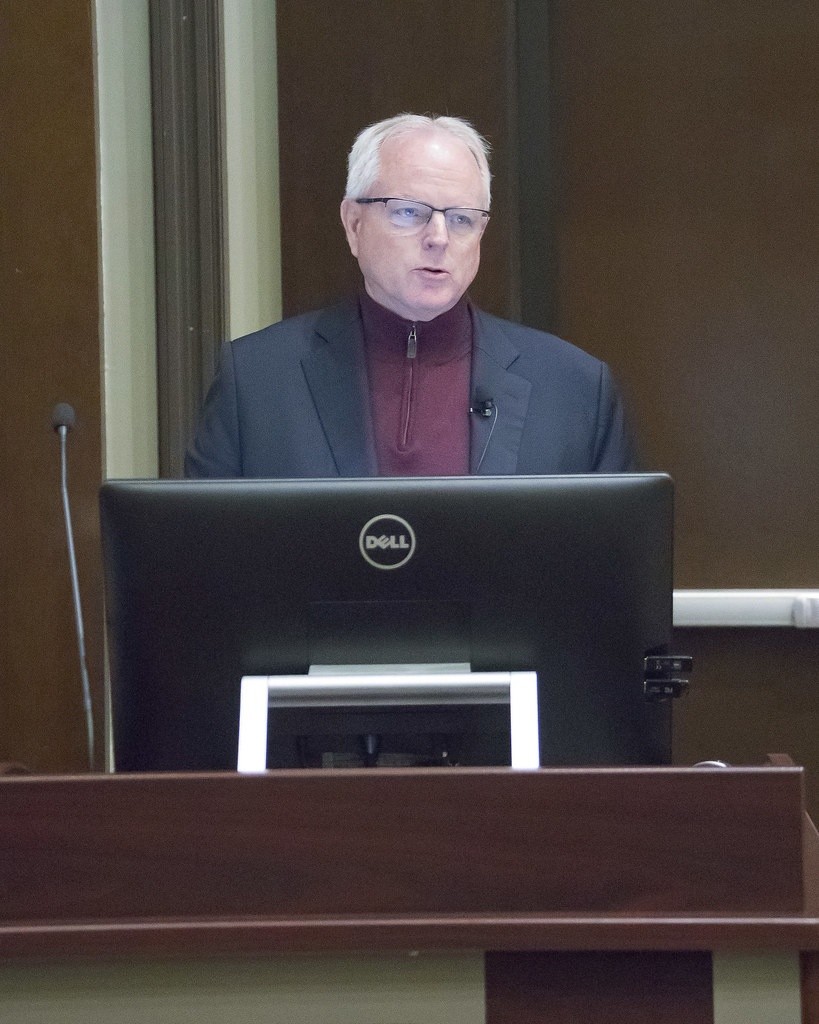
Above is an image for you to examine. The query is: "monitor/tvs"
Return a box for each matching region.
[97,472,678,777]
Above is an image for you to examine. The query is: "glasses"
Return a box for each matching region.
[355,196,489,243]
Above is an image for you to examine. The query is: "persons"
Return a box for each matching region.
[184,113,653,477]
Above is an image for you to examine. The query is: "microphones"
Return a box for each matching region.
[52,403,97,775]
[475,383,496,410]
